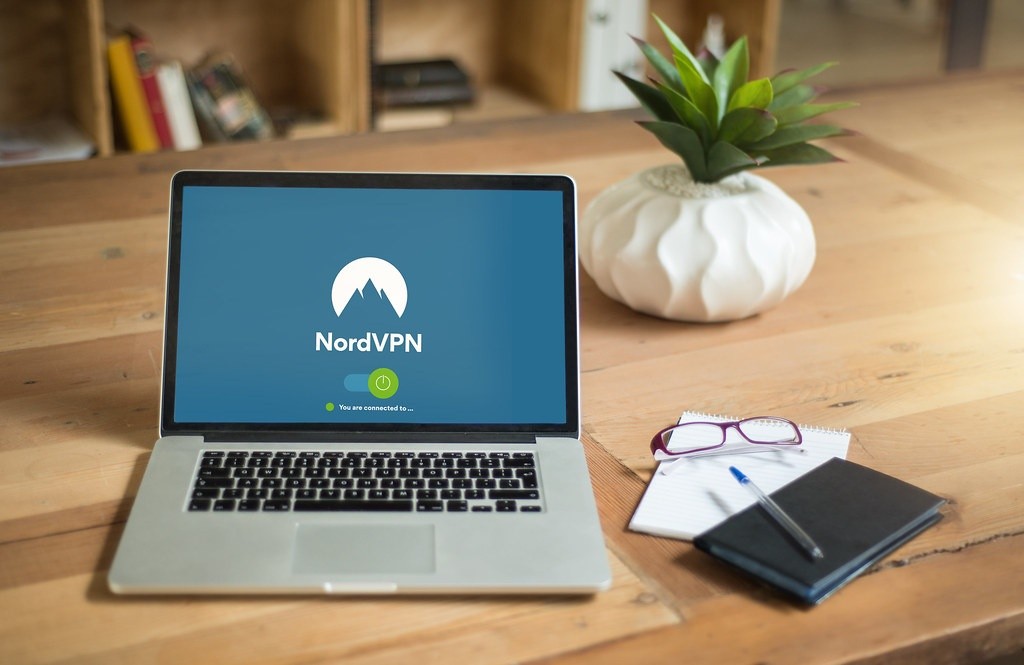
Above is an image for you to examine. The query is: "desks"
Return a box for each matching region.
[0,64,1024,665]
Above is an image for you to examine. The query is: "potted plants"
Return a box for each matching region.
[577,12,860,322]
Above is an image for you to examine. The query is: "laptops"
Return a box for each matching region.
[109,170,611,602]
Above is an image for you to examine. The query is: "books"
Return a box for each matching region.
[0,116,95,169]
[370,57,474,133]
[627,412,945,605]
[105,28,277,149]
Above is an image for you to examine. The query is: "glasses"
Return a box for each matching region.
[646,413,807,474]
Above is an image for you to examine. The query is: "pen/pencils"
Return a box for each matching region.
[727,460,826,565]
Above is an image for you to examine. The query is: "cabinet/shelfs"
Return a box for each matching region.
[0,0,782,163]
